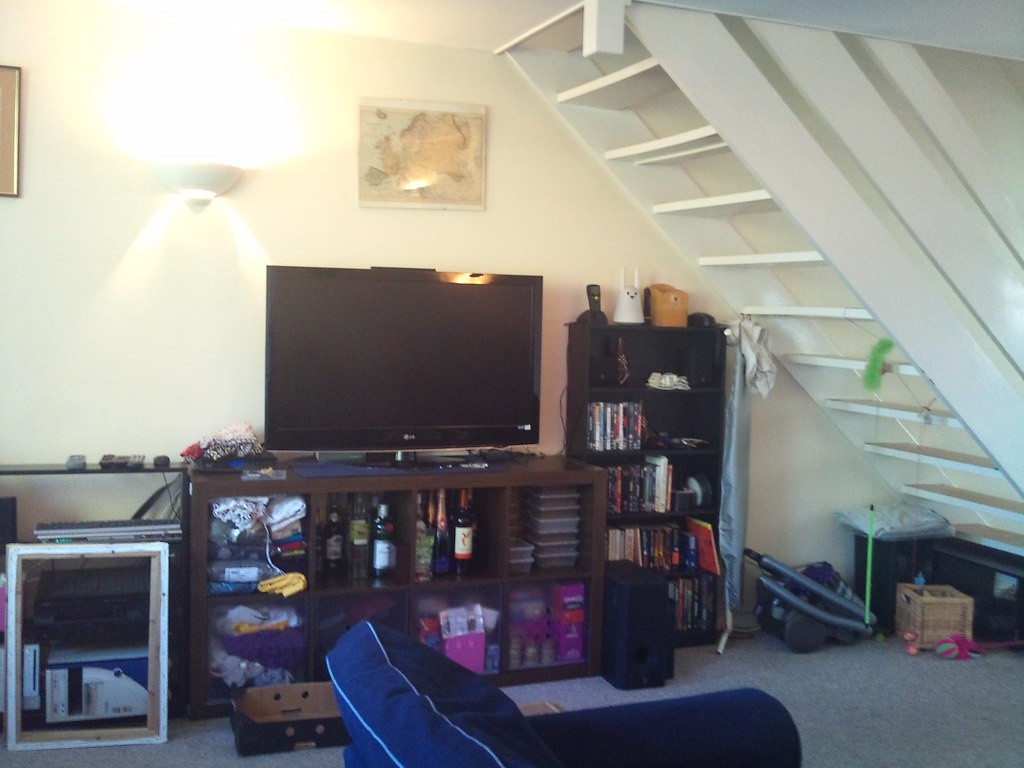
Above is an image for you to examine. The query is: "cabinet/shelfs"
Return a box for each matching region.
[566,322,726,648]
[0,466,188,729]
[184,464,610,719]
[855,532,1024,650]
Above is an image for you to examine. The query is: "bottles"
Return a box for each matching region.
[371,503,397,578]
[417,488,479,581]
[346,492,371,580]
[315,509,324,581]
[324,505,344,570]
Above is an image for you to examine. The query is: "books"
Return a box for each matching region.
[602,456,672,512]
[586,401,643,450]
[608,517,720,633]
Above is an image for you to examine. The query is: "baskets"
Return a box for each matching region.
[894,582,973,650]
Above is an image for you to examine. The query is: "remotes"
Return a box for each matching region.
[67,453,86,469]
[98,454,145,467]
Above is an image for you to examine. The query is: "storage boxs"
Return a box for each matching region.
[46,645,148,723]
[231,682,353,756]
[22,643,38,711]
[511,489,586,669]
[650,282,688,327]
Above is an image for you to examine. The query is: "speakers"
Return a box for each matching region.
[602,558,674,686]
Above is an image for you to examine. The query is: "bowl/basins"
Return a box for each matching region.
[509,488,581,575]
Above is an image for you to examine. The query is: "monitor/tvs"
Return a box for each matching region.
[262,265,543,469]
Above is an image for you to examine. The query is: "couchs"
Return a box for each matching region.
[325,621,803,768]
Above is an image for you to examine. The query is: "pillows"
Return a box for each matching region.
[834,502,954,545]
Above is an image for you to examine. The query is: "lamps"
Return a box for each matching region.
[171,161,242,198]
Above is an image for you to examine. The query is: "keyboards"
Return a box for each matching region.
[32,519,182,535]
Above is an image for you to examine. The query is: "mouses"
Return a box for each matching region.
[153,456,172,466]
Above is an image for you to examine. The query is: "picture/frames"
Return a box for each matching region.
[1,66,21,197]
[4,542,170,750]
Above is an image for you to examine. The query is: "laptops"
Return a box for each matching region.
[38,529,184,544]
